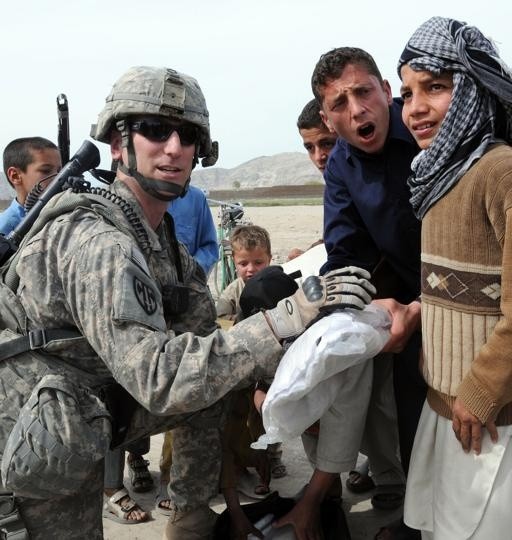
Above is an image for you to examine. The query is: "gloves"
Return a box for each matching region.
[266,266,377,337]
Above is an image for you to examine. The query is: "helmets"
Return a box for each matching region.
[91,66,213,156]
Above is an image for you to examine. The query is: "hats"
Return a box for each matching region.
[238,267,303,320]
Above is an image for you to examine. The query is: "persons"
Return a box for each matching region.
[1,136,61,237]
[298,97,406,509]
[1,65,377,540]
[309,46,430,540]
[395,15,512,540]
[101,183,352,540]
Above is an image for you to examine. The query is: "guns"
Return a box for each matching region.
[0,140,100,267]
[58,94,70,169]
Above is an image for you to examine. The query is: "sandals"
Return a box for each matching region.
[101,487,147,524]
[155,479,176,517]
[127,451,154,493]
[235,474,270,500]
[373,515,421,539]
[346,459,377,493]
[370,486,405,510]
[269,459,288,481]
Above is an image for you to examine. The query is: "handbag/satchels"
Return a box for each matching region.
[1,375,112,502]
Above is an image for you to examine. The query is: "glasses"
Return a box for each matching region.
[128,119,199,146]
